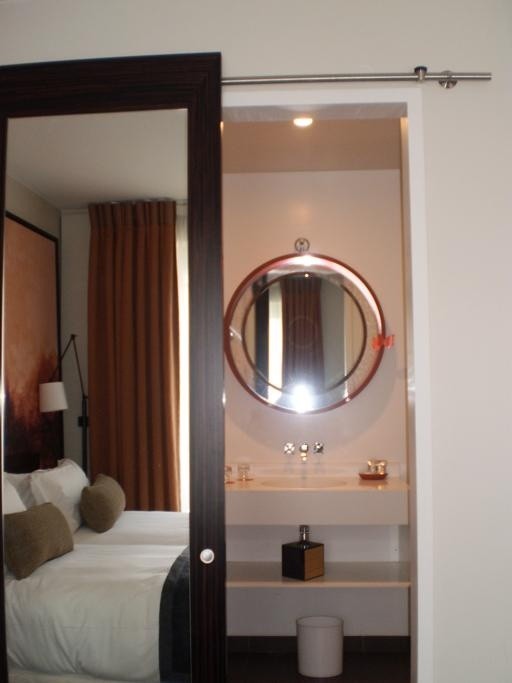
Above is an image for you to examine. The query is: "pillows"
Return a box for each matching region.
[80,473,126,533]
[2,469,43,515]
[29,458,91,535]
[3,502,74,581]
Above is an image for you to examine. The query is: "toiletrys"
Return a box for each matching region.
[281,525,326,581]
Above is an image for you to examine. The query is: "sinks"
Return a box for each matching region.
[262,476,352,488]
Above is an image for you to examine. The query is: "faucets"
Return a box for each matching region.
[299,442,309,463]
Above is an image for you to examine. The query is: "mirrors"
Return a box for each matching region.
[224,252,386,416]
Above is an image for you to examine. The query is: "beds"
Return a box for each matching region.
[1,449,191,683]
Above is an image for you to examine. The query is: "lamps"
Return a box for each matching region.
[37,332,88,473]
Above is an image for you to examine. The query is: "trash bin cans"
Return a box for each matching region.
[294,615,344,677]
